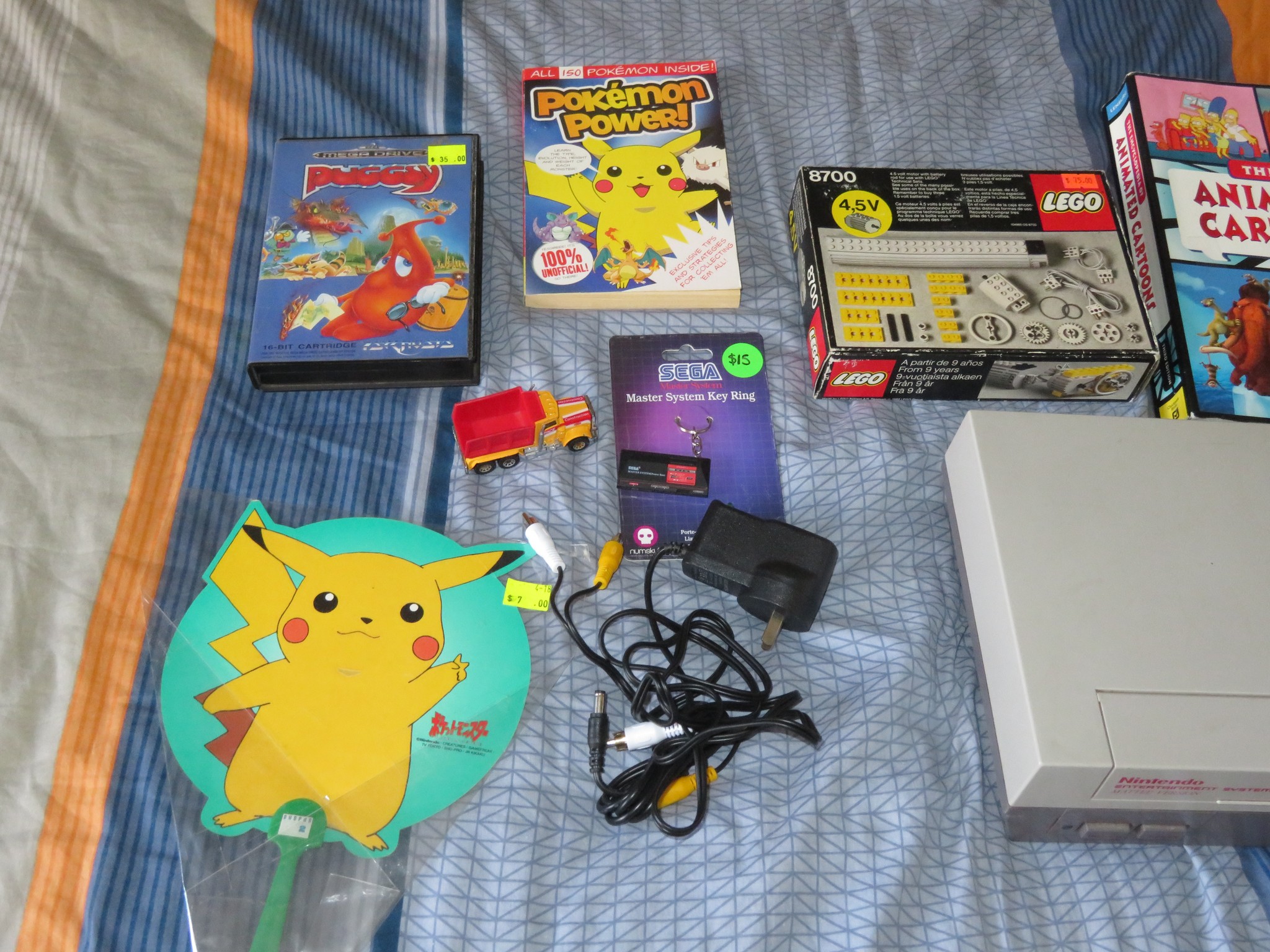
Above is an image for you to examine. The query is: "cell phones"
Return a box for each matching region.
[615,448,711,498]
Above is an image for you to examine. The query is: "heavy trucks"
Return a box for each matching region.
[451,385,599,475]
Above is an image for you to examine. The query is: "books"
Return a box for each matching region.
[1103,71,1270,424]
[521,60,742,308]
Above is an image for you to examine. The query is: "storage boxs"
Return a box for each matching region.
[788,164,1161,404]
